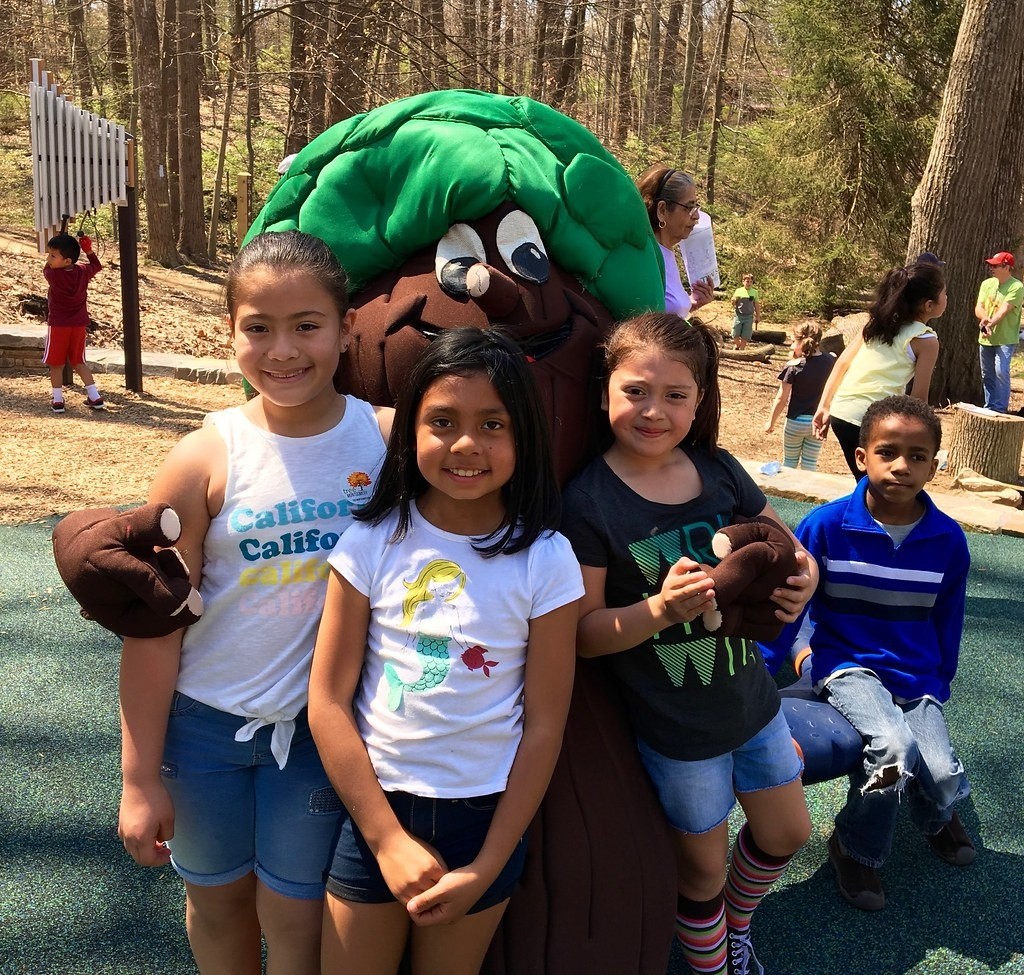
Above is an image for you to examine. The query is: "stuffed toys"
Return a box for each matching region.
[52,87,799,975]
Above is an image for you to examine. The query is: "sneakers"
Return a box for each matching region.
[828,828,886,912]
[727,925,764,975]
[50,397,66,413]
[922,810,976,865]
[82,396,105,410]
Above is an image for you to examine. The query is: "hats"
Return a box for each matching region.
[985,251,1015,267]
[917,252,947,267]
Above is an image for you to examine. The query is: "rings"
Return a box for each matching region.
[706,293,709,297]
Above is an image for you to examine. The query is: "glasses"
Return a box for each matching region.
[654,198,702,217]
[989,264,1006,269]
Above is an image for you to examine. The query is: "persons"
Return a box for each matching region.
[309,324,586,975]
[812,252,947,482]
[789,396,977,911]
[765,323,837,472]
[572,312,820,975]
[731,273,760,351]
[41,230,104,413]
[117,232,396,975]
[637,169,715,319]
[975,252,1024,414]
